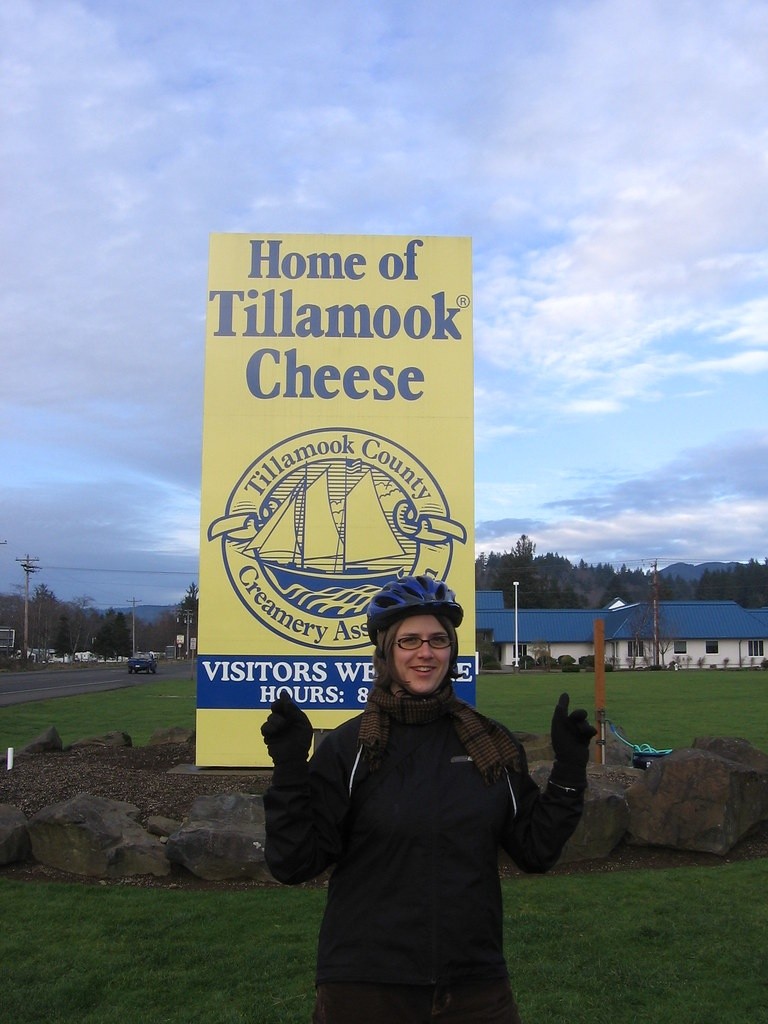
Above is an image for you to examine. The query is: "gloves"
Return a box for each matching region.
[260,685,314,787]
[546,691,599,795]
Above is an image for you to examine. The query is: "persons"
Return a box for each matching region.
[260,577,599,1024]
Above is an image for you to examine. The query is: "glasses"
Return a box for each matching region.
[391,635,456,651]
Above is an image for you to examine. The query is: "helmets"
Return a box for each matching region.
[366,577,464,646]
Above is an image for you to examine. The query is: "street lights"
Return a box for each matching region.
[513,582,520,668]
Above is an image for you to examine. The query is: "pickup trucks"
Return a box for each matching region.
[127,651,157,675]
